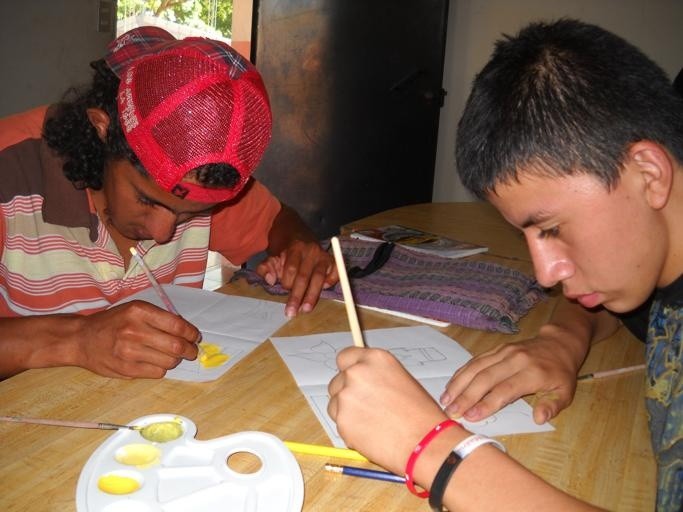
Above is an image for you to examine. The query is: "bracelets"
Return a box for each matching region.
[404,418,463,498]
[428,434,505,511]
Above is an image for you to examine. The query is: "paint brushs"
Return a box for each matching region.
[576,364,647,380]
[0,416,143,430]
[129,247,208,363]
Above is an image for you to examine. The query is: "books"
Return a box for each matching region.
[348,225,488,261]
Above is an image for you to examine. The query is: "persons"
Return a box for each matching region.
[0,27,341,381]
[328,23,681,511]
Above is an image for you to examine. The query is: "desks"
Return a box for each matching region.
[0,201,658,511]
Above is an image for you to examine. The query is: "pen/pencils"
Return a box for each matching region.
[283,441,370,461]
[321,463,416,485]
[331,236,365,347]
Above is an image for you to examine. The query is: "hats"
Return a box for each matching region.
[104,26,273,203]
